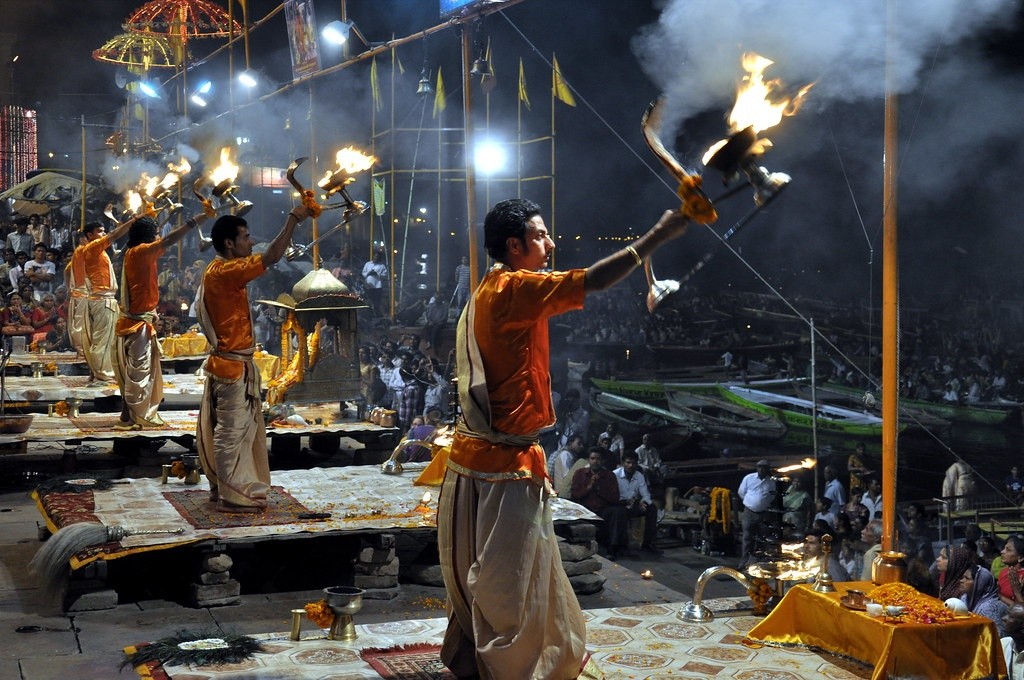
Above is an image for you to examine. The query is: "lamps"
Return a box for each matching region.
[239,68,259,87]
[323,21,354,45]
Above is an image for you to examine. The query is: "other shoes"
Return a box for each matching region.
[605,547,617,562]
[217,498,261,513]
[641,542,665,554]
[209,489,219,502]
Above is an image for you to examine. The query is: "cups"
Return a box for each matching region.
[846,589,866,605]
[866,603,884,617]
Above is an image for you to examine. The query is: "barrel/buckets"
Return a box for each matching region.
[327,615,356,641]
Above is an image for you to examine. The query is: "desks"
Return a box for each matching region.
[745,579,1008,679]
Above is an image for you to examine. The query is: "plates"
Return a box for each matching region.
[840,597,879,610]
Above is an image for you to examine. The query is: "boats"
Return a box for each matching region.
[715,380,909,439]
[591,378,724,403]
[589,389,698,459]
[822,382,1014,427]
[665,385,788,438]
[791,378,953,434]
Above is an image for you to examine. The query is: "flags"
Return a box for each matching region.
[370,35,577,117]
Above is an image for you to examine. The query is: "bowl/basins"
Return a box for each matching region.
[0,414,35,433]
[82,466,124,479]
[323,586,366,615]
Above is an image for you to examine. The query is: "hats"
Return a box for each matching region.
[756,459,770,468]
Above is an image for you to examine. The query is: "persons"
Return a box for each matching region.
[435,198,690,680]
[161,213,480,462]
[0,190,210,429]
[547,261,1024,680]
[193,205,312,511]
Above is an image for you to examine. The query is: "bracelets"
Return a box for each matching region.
[134,216,139,220]
[186,218,196,228]
[289,213,300,223]
